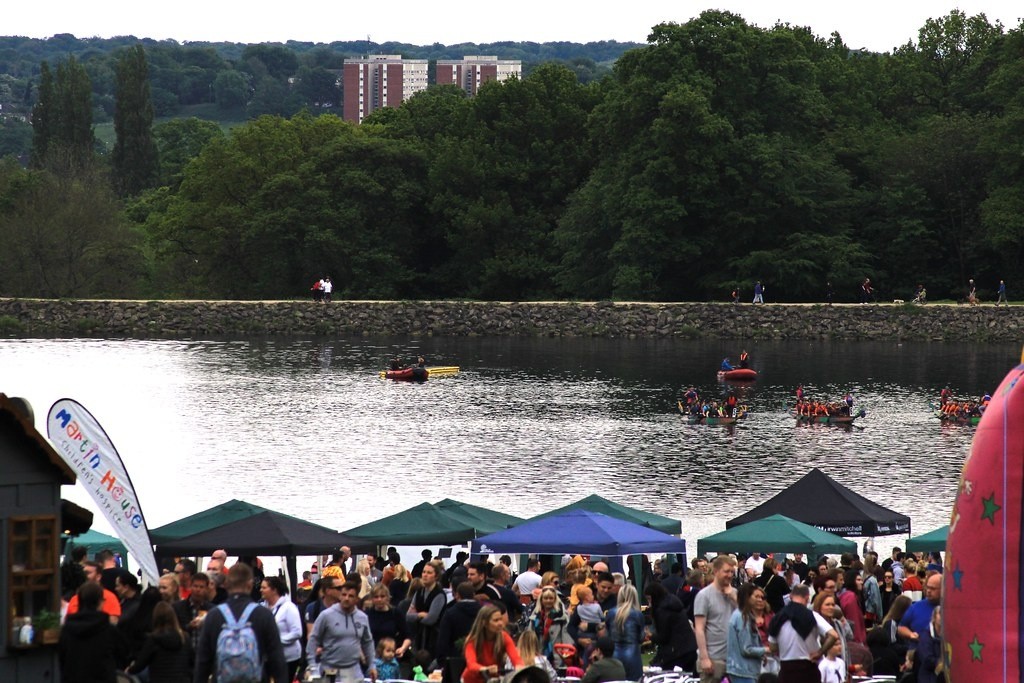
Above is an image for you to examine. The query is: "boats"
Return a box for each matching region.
[384,367,429,379]
[718,369,758,379]
[674,401,747,425]
[929,396,981,426]
[789,409,866,422]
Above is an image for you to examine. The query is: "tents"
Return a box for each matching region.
[60,468,949,613]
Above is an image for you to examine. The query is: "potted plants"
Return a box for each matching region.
[32,607,62,644]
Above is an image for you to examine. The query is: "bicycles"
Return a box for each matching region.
[910,292,927,305]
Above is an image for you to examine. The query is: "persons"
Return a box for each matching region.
[863,277,871,305]
[797,384,806,404]
[861,283,866,304]
[740,349,749,370]
[940,385,992,423]
[994,280,1008,306]
[912,285,927,305]
[795,398,849,418]
[826,280,833,304]
[720,356,735,372]
[416,355,425,367]
[685,386,698,411]
[969,279,978,305]
[60,538,945,683]
[732,288,739,303]
[687,392,748,420]
[844,391,854,416]
[752,281,765,304]
[389,356,400,371]
[312,277,333,302]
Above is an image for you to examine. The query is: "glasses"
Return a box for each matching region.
[591,570,603,575]
[884,576,893,579]
[211,556,222,559]
[208,567,220,572]
[857,579,863,582]
[175,570,185,574]
[922,584,943,592]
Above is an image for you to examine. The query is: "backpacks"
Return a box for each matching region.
[216,601,262,683]
